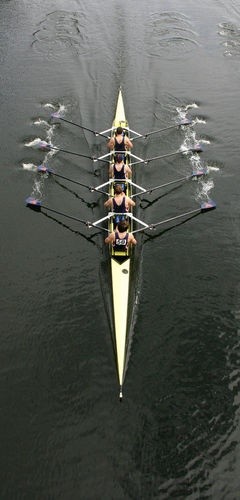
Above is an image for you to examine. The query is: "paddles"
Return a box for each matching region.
[31,166,113,198]
[129,119,194,140]
[130,167,209,199]
[40,143,112,164]
[130,201,216,234]
[50,112,111,140]
[25,197,112,233]
[129,143,205,166]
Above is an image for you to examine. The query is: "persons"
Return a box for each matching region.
[107,126,133,154]
[105,223,136,252]
[104,185,136,216]
[109,154,132,182]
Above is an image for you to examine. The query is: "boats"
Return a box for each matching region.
[105,90,133,400]
